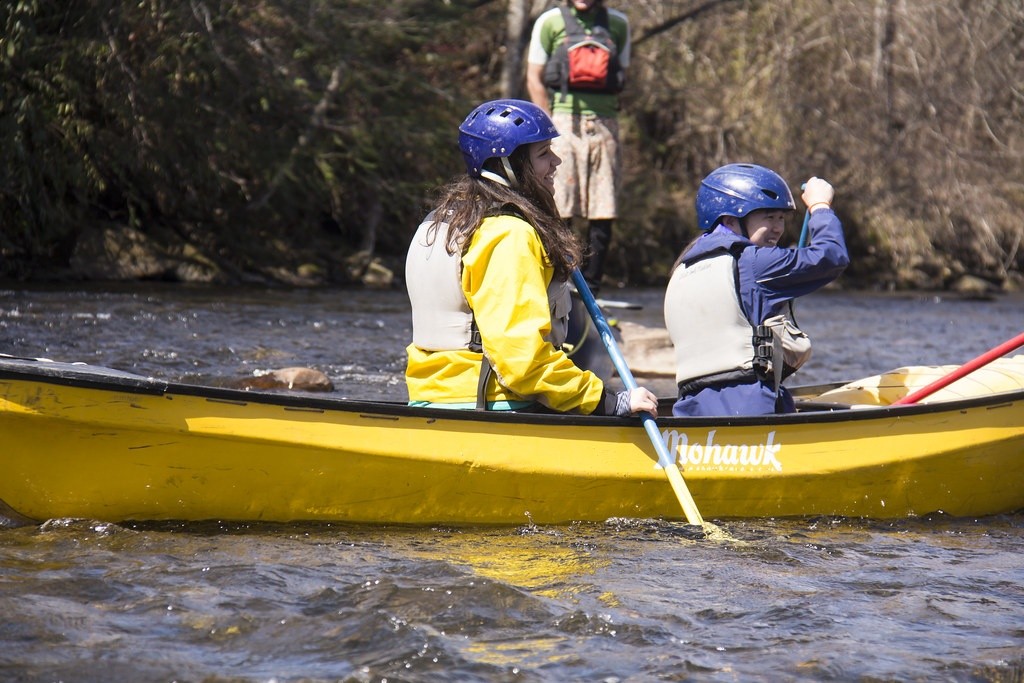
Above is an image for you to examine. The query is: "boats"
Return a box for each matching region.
[1,352,1023,522]
[609,315,673,375]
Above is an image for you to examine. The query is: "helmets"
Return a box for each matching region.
[694,163,797,230]
[459,98,559,179]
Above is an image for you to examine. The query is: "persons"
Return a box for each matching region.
[665,163,850,417]
[405,99,659,417]
[527,0,632,300]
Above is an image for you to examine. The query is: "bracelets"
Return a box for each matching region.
[809,201,831,212]
